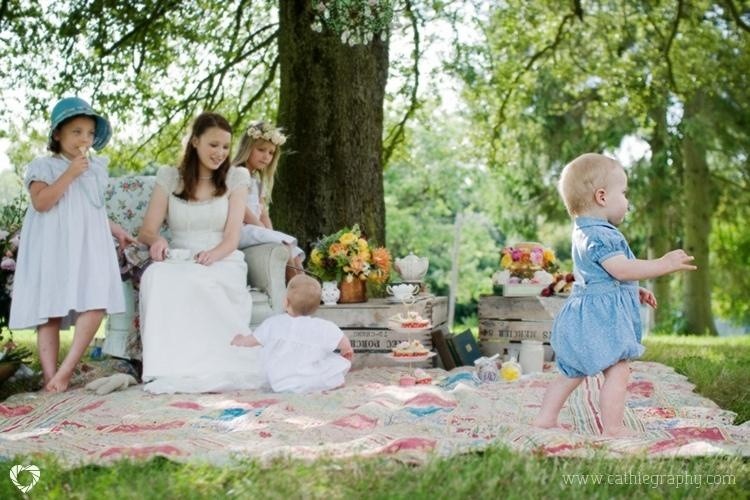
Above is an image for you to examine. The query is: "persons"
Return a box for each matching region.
[233,120,305,281]
[230,272,354,397]
[530,152,699,440]
[9,99,136,395]
[137,110,250,386]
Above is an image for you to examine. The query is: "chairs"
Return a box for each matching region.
[100,175,291,361]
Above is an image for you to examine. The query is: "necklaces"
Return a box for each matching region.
[197,174,214,180]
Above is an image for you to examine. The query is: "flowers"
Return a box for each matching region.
[0,191,31,364]
[492,240,560,286]
[248,120,287,146]
[308,224,389,289]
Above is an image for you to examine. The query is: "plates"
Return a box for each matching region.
[164,258,197,264]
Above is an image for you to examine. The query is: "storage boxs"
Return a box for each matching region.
[319,296,448,371]
[479,296,568,363]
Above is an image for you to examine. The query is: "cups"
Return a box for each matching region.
[164,248,189,260]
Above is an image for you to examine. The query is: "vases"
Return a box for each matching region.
[502,286,545,297]
[332,274,369,303]
[1,361,20,390]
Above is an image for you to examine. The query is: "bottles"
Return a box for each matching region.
[519,340,543,374]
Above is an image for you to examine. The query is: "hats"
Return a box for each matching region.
[47,97,112,152]
